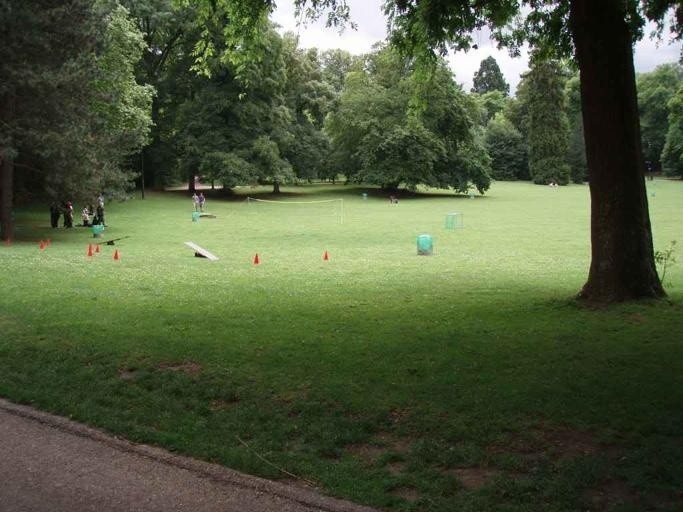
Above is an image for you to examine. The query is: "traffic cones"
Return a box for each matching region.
[324,251,329,260]
[113,249,119,259]
[254,252,259,264]
[88,243,93,256]
[40,239,51,249]
[95,244,100,253]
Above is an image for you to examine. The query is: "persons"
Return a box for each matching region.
[46,191,108,229]
[198,192,205,212]
[191,191,199,212]
